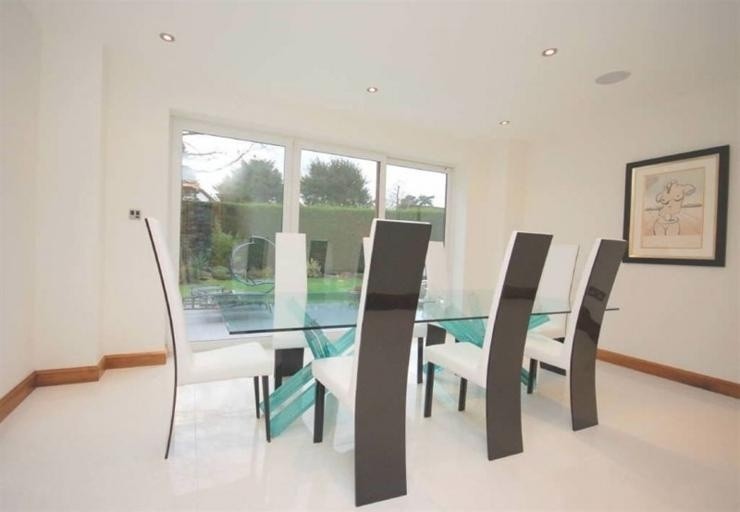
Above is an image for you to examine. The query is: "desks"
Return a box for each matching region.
[209,280,620,444]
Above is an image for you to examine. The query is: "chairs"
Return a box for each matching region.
[272,230,307,388]
[360,235,423,386]
[538,240,579,376]
[147,219,276,459]
[425,230,553,460]
[311,217,434,508]
[423,241,465,347]
[525,237,627,432]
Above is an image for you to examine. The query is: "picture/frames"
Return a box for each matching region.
[622,143,731,267]
